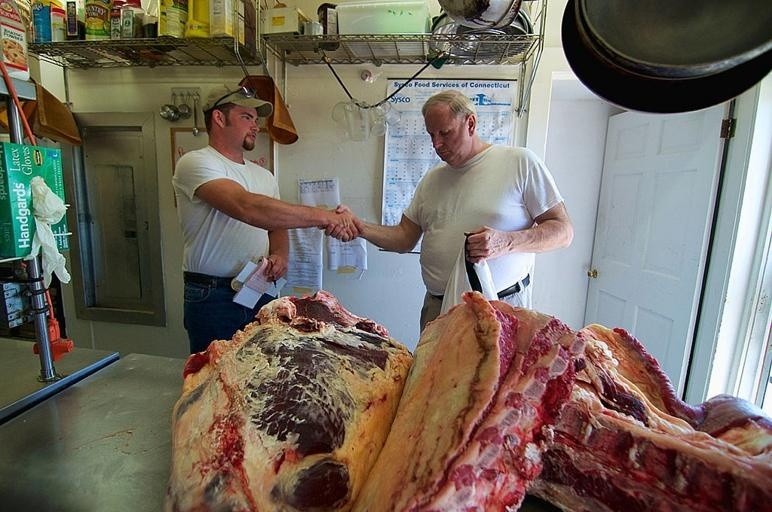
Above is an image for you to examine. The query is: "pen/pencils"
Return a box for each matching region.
[271,261,276,288]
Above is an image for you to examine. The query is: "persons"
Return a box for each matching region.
[313,89,574,333]
[170,82,368,357]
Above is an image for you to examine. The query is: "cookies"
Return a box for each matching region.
[2,39,27,66]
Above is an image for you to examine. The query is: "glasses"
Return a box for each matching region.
[215,86,258,106]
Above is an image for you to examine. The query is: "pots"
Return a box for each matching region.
[560,1,771,121]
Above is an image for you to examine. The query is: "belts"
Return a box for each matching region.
[432,272,531,300]
[183,270,235,288]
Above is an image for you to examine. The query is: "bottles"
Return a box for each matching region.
[119,1,144,38]
[109,1,125,38]
[51,7,68,41]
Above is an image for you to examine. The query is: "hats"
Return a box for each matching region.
[202,82,273,118]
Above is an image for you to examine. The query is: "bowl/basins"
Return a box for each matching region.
[429,2,536,63]
